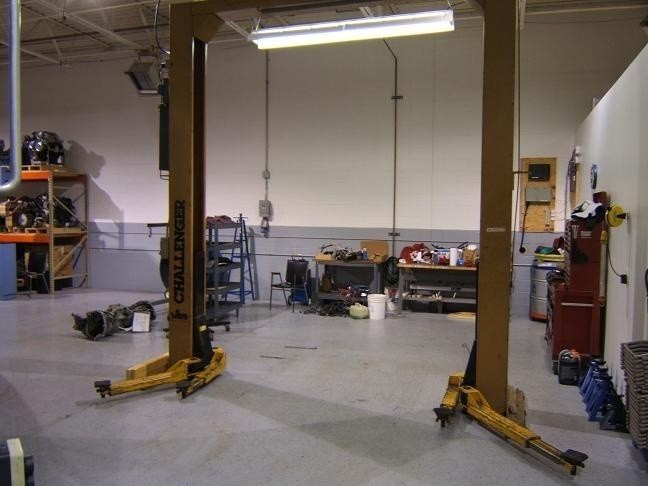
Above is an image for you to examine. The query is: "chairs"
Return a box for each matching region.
[269,255,312,313]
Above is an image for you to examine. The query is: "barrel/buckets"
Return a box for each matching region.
[529,260,565,322]
[557,348,581,386]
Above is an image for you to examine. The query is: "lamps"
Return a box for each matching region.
[248,0,456,50]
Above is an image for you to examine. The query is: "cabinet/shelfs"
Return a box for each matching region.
[544,191,607,376]
[166,219,245,332]
[0,165,89,295]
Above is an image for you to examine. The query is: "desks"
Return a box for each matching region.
[396,262,477,314]
[315,252,386,313]
